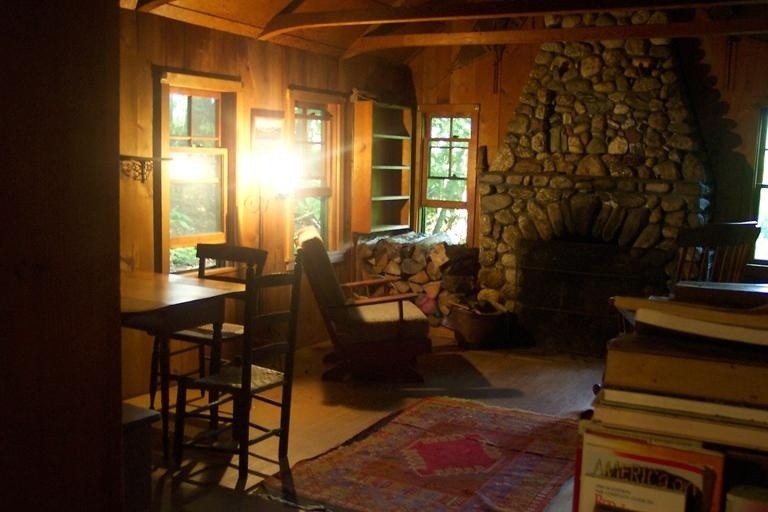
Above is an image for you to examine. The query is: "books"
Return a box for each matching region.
[572,296,768,512]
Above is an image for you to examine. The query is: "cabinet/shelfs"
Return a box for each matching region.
[350,100,411,233]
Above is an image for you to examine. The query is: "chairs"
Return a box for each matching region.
[157,243,270,440]
[178,259,305,481]
[591,218,763,396]
[292,225,433,385]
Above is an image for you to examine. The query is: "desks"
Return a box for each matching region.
[119,266,249,476]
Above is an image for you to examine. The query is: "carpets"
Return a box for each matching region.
[249,392,589,510]
